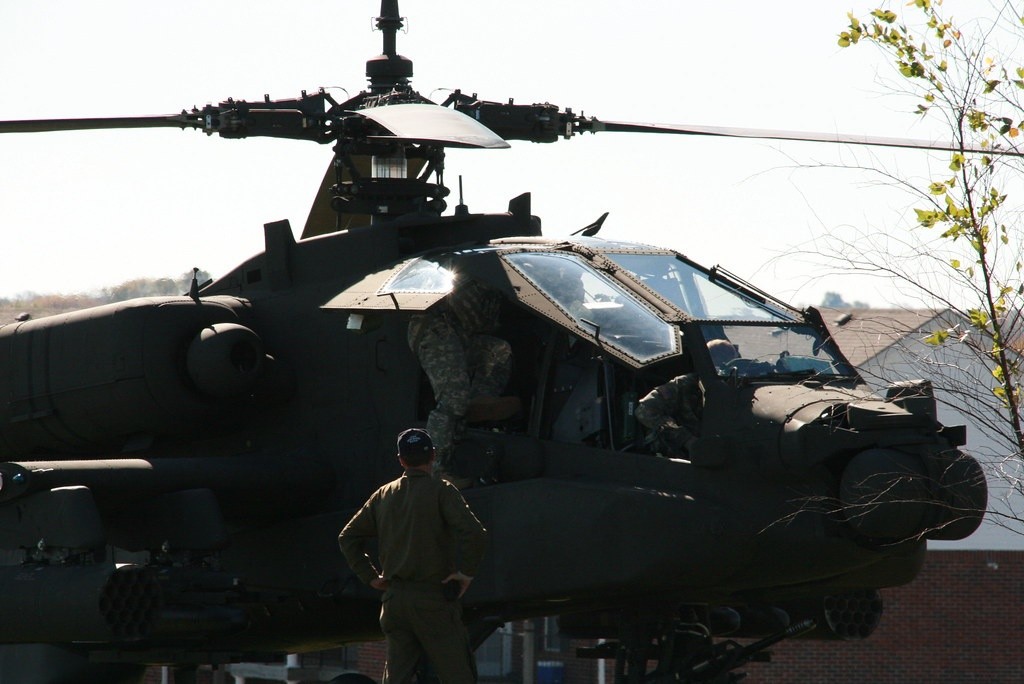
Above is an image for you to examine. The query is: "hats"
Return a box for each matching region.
[706,339,739,368]
[397,428,434,455]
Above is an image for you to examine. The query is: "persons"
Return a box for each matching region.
[635,339,738,456]
[408,271,522,489]
[339,429,487,684]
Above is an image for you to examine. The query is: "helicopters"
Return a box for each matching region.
[0,0,1024,684]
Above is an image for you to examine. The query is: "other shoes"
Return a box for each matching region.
[468,397,522,421]
[438,470,470,489]
